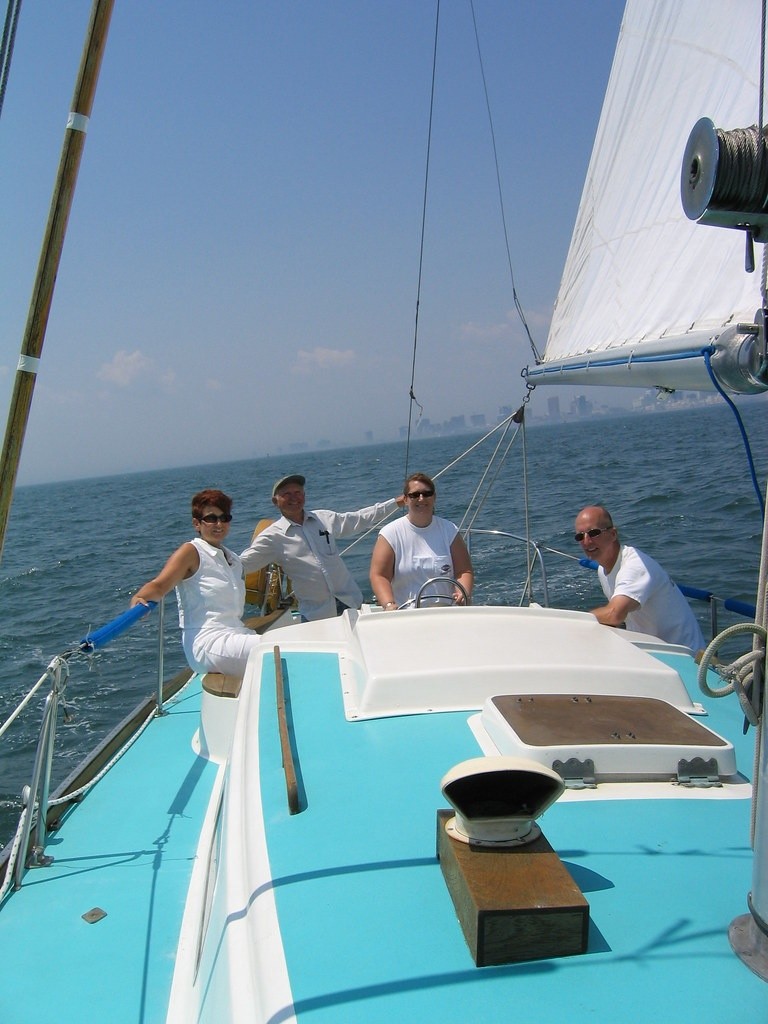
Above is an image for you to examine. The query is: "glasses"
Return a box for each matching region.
[198,513,232,523]
[575,525,613,541]
[406,490,435,499]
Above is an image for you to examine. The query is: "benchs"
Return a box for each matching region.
[191,606,302,765]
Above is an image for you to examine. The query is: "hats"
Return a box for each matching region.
[272,474,306,497]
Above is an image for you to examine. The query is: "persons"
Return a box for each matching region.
[371,474,474,612]
[131,489,266,677]
[240,475,406,622]
[575,506,708,658]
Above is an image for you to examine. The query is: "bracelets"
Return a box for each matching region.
[382,601,396,609]
